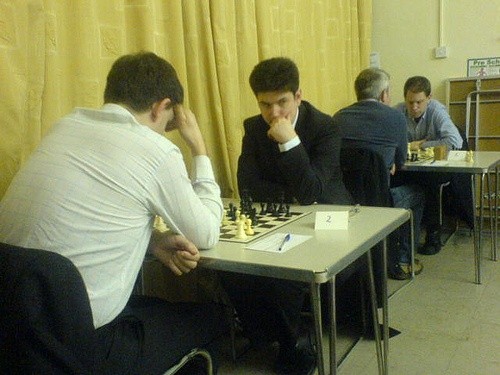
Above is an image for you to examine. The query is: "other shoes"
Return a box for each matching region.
[273,323,318,375]
[422,228,441,256]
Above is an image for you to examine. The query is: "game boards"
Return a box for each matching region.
[153,205,312,243]
[405,149,435,164]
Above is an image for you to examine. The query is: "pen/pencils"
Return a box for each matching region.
[279,233,291,251]
[430,160,436,164]
[349,204,360,217]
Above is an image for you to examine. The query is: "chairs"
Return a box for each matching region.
[143,197,410,375]
[0,127,477,375]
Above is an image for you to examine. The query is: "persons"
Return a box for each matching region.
[216,57,361,375]
[389,76,464,255]
[0,50,224,375]
[333,66,424,281]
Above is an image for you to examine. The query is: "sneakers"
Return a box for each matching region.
[395,259,423,279]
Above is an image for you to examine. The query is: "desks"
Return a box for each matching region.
[397,150,500,284]
